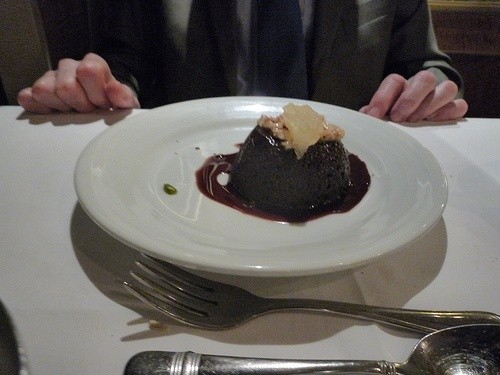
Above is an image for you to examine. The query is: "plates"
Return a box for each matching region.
[73,96,449,277]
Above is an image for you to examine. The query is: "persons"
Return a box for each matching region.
[17,0,470,125]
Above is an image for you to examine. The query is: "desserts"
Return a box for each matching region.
[227,101,352,217]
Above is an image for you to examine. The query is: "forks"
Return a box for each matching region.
[122,251,499,337]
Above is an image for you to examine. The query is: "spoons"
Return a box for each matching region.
[123,322,500,375]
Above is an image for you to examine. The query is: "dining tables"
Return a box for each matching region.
[0,99,500,375]
